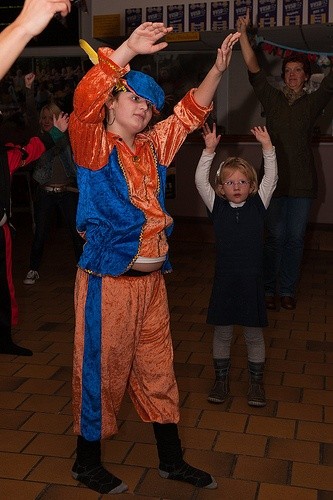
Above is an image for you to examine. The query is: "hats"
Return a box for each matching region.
[121,70,165,113]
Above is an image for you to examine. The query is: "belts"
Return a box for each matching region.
[40,184,80,194]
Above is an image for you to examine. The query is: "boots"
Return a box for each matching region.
[247,359,266,405]
[207,358,232,403]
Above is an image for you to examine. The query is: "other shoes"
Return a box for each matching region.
[281,296,297,308]
[264,295,276,309]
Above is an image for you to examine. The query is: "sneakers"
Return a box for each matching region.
[22,270,40,284]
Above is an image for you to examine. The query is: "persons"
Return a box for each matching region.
[24,73,86,284]
[68,22,241,495]
[0,66,83,111]
[236,8,333,310]
[0,111,70,356]
[0,0,71,80]
[195,123,278,407]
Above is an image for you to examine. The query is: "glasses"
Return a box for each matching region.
[223,180,250,189]
